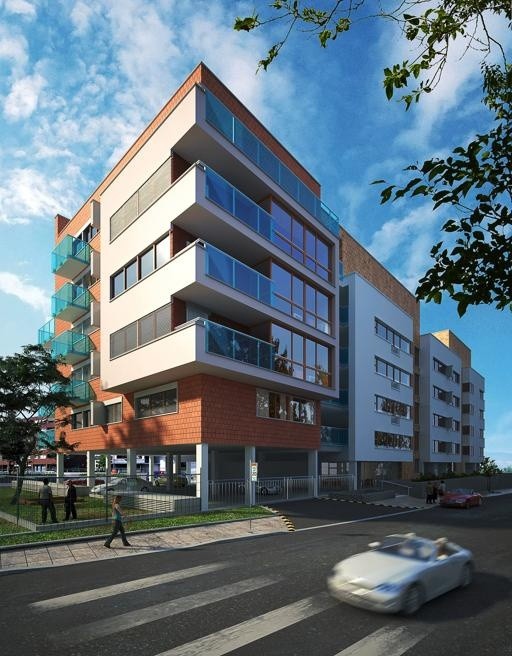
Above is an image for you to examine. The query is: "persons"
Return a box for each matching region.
[62,480,77,521]
[104,495,131,548]
[435,537,449,560]
[425,480,446,504]
[38,477,58,524]
